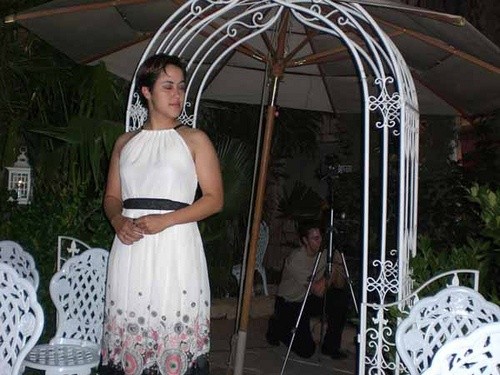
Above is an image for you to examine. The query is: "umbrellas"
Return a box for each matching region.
[4,0,500,375]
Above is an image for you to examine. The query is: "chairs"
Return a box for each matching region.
[394,286,500,375]
[231,219,269,296]
[0,240,109,375]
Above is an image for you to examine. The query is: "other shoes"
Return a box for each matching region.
[267,317,281,347]
[321,345,348,359]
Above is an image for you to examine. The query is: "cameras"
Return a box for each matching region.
[320,160,353,175]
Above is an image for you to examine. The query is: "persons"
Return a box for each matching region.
[265,220,351,360]
[97,53,226,375]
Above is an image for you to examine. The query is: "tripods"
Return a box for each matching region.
[281,177,358,375]
